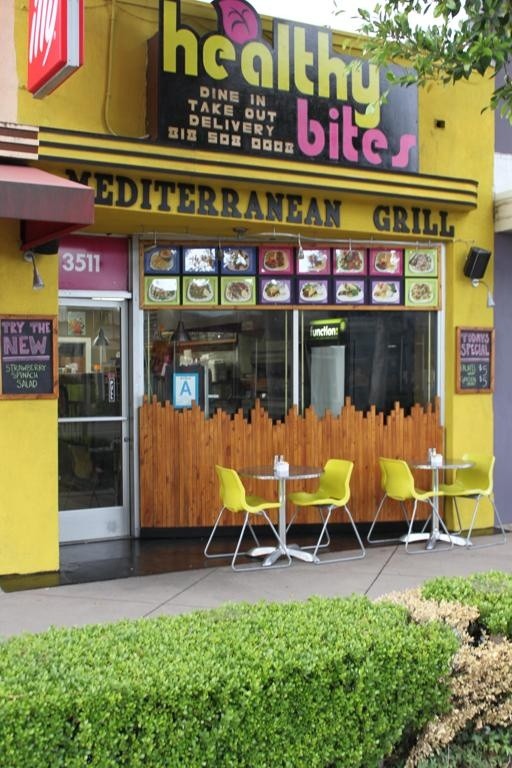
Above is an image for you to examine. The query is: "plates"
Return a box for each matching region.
[149,248,435,304]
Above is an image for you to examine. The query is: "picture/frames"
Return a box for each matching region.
[66,309,87,337]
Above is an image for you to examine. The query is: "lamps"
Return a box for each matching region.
[93,328,111,372]
[23,250,44,288]
[170,310,191,342]
[472,278,495,308]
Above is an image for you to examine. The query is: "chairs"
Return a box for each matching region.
[68,445,103,509]
[367,456,453,555]
[287,458,366,565]
[439,453,507,550]
[203,465,292,572]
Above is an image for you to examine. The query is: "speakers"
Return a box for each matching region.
[30,238,58,254]
[464,246,491,278]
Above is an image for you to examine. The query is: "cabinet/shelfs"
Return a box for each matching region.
[144,323,239,419]
[236,309,310,424]
[58,335,92,449]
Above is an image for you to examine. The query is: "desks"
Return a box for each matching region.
[409,460,476,550]
[90,447,115,454]
[237,466,325,567]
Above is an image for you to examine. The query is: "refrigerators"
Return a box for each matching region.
[305,316,414,419]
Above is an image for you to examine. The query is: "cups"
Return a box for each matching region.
[274,462,289,471]
[432,454,443,466]
